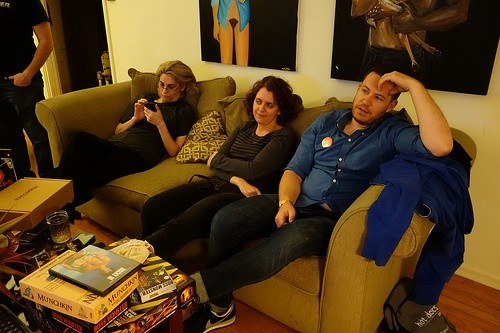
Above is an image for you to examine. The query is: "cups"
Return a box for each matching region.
[45,209,71,243]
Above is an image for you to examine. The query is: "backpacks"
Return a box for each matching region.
[377,276,458,333]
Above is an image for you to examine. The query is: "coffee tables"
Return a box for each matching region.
[0,222,199,333]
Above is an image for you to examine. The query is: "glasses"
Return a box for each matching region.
[156,82,181,93]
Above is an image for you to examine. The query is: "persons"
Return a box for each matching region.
[55,60,200,219]
[141,77,301,260]
[181,67,453,333]
[0,0,54,179]
[60,250,115,276]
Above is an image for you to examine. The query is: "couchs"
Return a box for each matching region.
[35,68,477,333]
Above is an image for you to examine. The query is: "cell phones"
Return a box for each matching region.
[71,233,96,247]
[145,102,157,112]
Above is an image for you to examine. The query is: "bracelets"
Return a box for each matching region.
[278,200,293,207]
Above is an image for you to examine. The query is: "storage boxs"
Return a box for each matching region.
[0,177,75,235]
[19,236,196,333]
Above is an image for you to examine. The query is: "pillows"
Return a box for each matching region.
[176,111,228,163]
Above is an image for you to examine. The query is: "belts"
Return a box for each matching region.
[310,203,338,221]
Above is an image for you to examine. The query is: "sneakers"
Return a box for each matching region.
[184,299,238,333]
